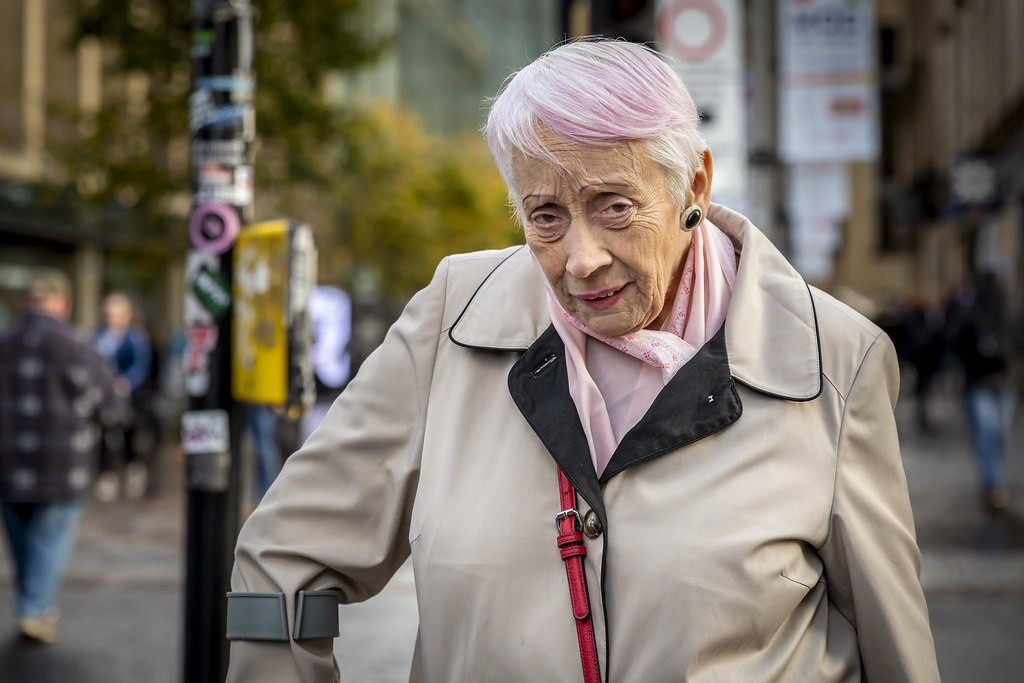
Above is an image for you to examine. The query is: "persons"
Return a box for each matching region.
[951,274,1016,510]
[225,41,940,683]
[0,268,151,645]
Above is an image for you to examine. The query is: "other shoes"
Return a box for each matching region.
[983,487,1012,511]
[16,616,59,644]
